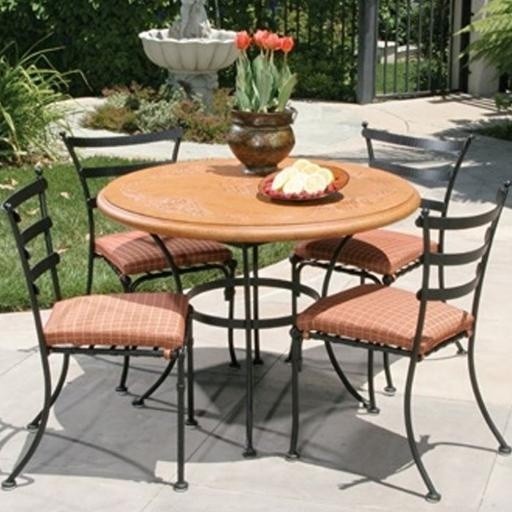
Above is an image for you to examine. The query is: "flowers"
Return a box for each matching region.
[233,28,298,113]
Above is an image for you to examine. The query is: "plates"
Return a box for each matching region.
[258,165,349,204]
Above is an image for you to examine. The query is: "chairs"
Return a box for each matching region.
[284,179,511,500]
[3,163,198,492]
[280,121,476,394]
[61,124,240,389]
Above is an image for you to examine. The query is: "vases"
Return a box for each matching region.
[227,108,295,177]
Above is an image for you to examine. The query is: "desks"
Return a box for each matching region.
[95,156,420,459]
